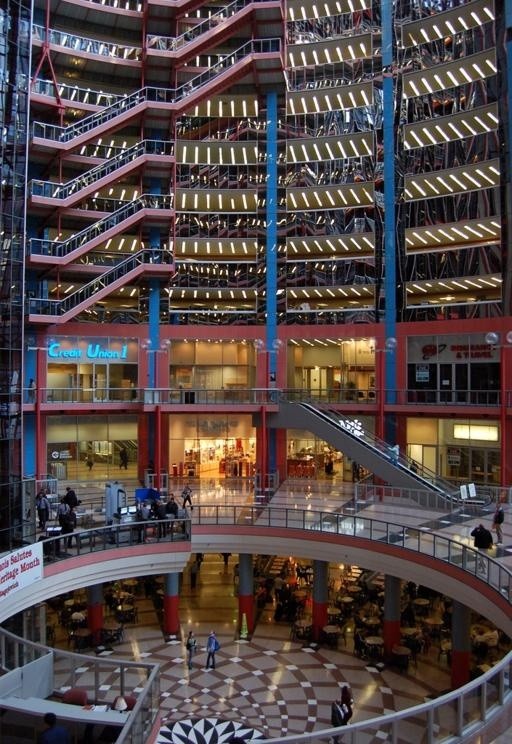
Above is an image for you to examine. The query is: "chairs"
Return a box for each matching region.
[62,689,87,706]
[111,696,136,710]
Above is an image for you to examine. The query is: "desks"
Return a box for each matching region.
[54,575,166,650]
[294,586,495,673]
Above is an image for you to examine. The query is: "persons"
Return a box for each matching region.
[71,605,85,622]
[340,685,352,725]
[295,562,308,582]
[187,630,196,669]
[470,522,492,574]
[36,479,193,548]
[493,502,504,545]
[37,712,71,744]
[196,552,204,570]
[119,446,128,469]
[25,378,37,404]
[220,552,232,564]
[274,574,284,600]
[351,461,360,482]
[330,698,345,744]
[87,449,94,471]
[205,629,219,669]
[410,460,418,473]
[189,560,198,588]
